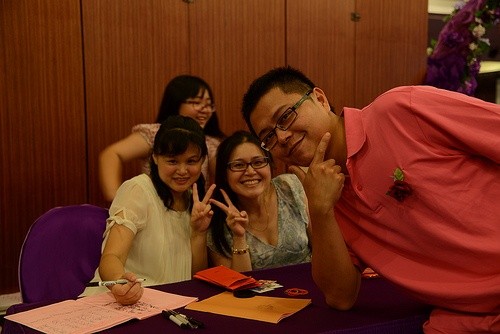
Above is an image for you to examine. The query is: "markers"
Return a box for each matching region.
[169,310,192,327]
[81,279,146,287]
[178,312,202,328]
[162,310,187,328]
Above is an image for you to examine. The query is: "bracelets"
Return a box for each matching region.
[231,247,249,255]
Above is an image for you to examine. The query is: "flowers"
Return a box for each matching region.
[427,0,500,86]
[385,166,414,202]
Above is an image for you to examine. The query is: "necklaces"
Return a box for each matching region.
[249,205,270,232]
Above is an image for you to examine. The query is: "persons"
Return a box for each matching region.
[98,75,226,209]
[241,66,500,334]
[78,115,218,305]
[206,131,315,272]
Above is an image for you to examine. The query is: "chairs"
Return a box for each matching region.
[17,205,110,302]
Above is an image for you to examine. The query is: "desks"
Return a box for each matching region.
[0,263,430,334]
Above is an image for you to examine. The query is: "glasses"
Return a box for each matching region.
[260,90,313,152]
[226,157,271,172]
[183,100,216,113]
